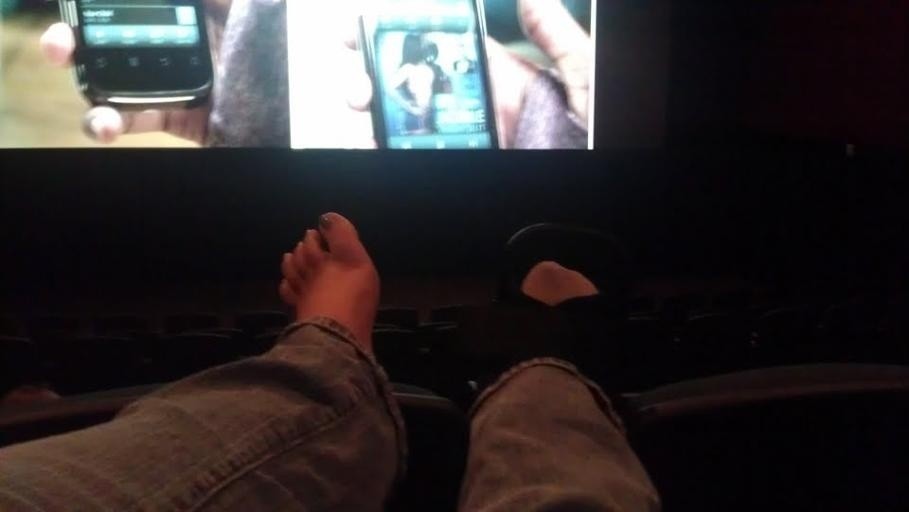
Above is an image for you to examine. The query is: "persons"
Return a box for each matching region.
[0,212,662,512]
[42,0,290,150]
[386,29,451,135]
[331,1,596,151]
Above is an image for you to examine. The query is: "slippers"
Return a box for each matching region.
[491,222,635,405]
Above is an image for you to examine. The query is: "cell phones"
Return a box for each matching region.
[358,0,503,148]
[58,0,214,108]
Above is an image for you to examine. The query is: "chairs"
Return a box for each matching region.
[13,296,909,512]
